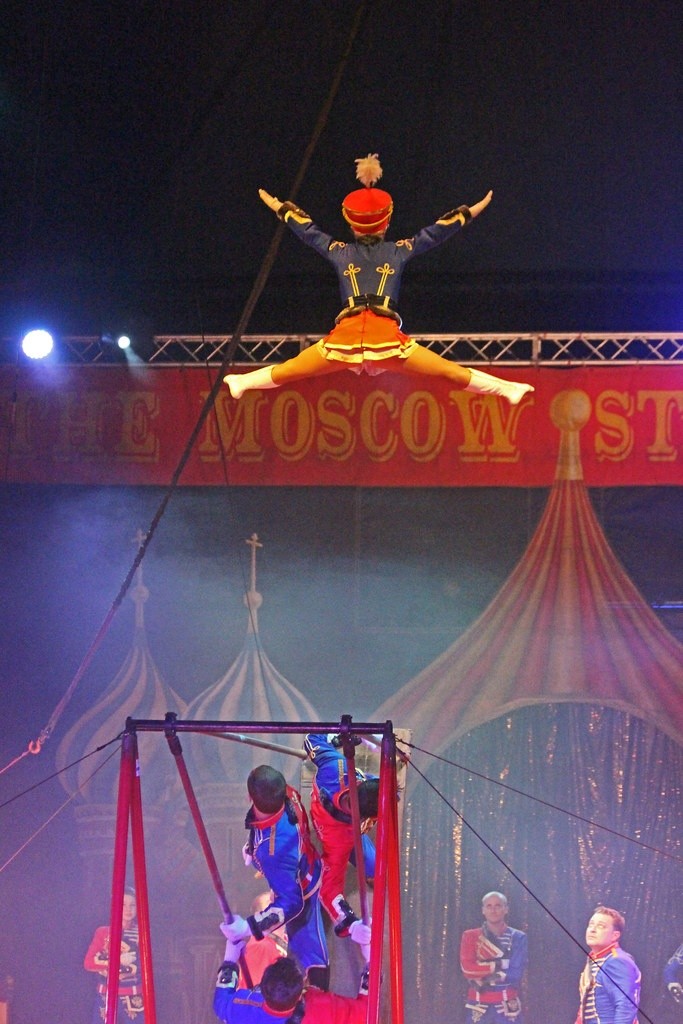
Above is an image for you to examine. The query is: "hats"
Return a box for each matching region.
[342,152,393,235]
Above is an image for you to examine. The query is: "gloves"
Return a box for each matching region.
[469,191,493,217]
[221,937,246,962]
[357,943,371,964]
[348,916,372,945]
[259,189,284,212]
[220,914,253,942]
[241,842,255,865]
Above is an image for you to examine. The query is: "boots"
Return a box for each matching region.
[223,365,280,399]
[463,368,535,405]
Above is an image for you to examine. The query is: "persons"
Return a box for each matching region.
[237,893,289,990]
[460,890,528,1024]
[212,939,382,1024]
[223,186,534,406]
[663,943,683,1007]
[303,725,406,944]
[82,885,147,1024]
[574,907,642,1024]
[218,763,331,989]
